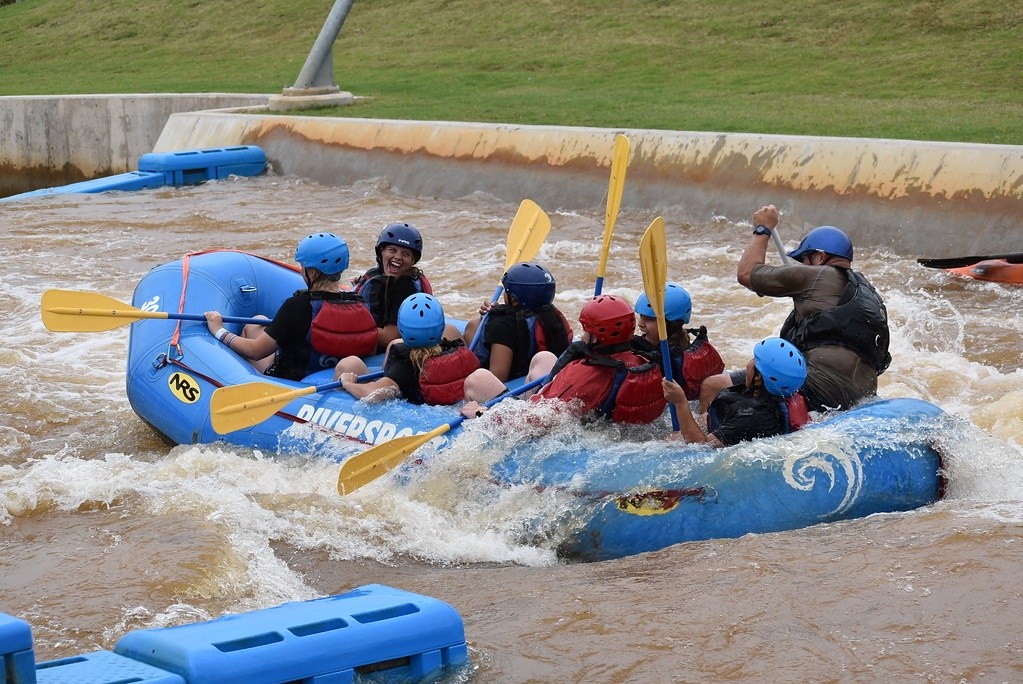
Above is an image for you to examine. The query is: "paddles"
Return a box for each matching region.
[593,132,631,296]
[462,197,552,354]
[210,371,385,434]
[771,227,789,264]
[339,373,553,497]
[39,290,275,335]
[639,214,680,436]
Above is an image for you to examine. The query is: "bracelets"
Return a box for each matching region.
[227,334,237,348]
[215,328,230,341]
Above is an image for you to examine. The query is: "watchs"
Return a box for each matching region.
[752,224,771,239]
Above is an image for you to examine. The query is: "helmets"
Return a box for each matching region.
[502,262,556,311]
[634,283,692,324]
[397,292,445,348]
[753,337,807,399]
[377,222,422,265]
[294,232,350,274]
[787,226,853,262]
[579,295,636,345]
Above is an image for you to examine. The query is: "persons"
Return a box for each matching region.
[350,221,432,343]
[634,282,694,390]
[441,263,574,384]
[696,202,878,416]
[663,337,806,448]
[332,293,480,406]
[202,232,377,382]
[459,293,642,419]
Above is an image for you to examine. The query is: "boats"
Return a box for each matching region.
[127,249,948,567]
